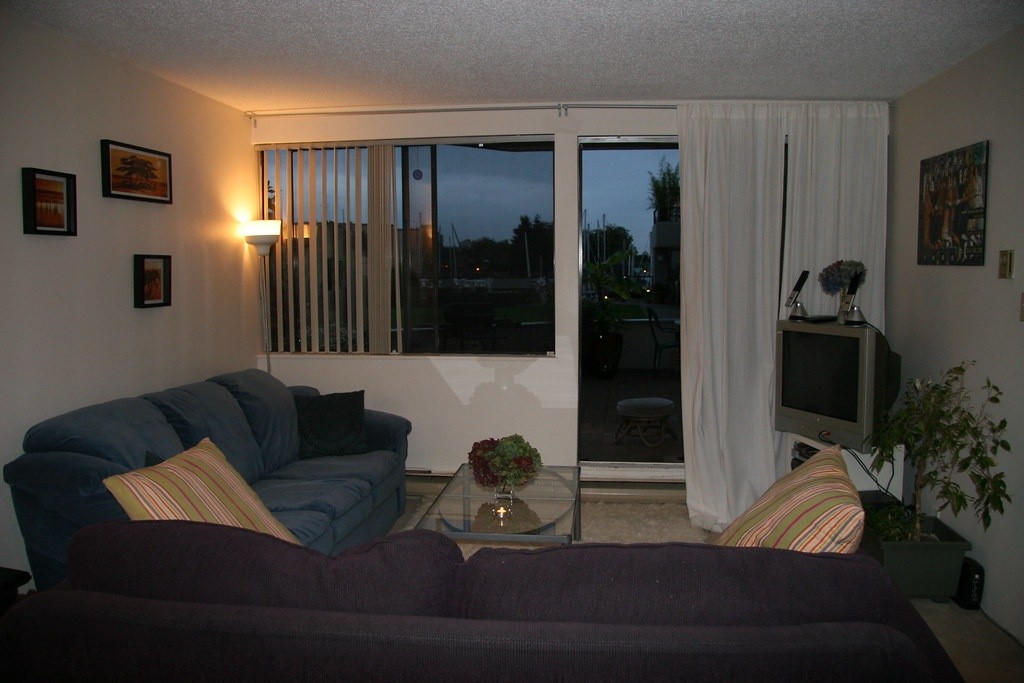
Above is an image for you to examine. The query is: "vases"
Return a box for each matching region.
[494,480,513,500]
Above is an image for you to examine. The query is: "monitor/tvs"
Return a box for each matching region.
[773,319,901,454]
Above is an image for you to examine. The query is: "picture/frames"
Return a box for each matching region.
[133,253,172,309]
[100,139,173,205]
[21,167,79,237]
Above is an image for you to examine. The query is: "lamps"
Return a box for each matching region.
[242,218,282,375]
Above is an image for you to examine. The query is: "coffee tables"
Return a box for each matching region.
[414,461,582,548]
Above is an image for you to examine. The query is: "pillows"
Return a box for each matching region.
[714,443,865,554]
[105,436,299,549]
[292,389,371,460]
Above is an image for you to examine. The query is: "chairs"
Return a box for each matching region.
[647,306,680,373]
[439,300,515,352]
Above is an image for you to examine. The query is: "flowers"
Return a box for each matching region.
[467,433,543,489]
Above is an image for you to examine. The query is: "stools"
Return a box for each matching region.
[612,398,678,447]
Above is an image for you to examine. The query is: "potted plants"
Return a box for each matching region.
[863,357,1012,594]
[580,249,644,381]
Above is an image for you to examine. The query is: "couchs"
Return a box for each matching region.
[3,368,412,589]
[0,518,967,682]
